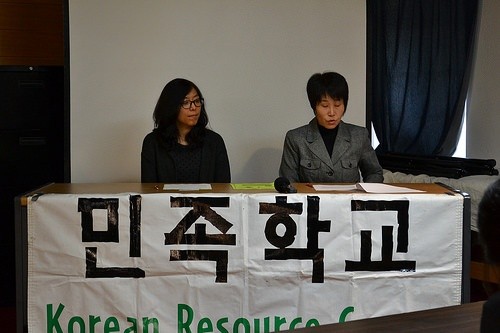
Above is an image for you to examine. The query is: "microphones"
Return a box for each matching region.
[275,177,297,193]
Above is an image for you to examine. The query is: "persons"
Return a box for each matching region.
[277,70,386,183]
[140,76,231,183]
[478,179,500,333]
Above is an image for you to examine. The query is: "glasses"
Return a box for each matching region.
[181,97,204,110]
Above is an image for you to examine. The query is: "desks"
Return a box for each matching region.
[15,182,500,333]
